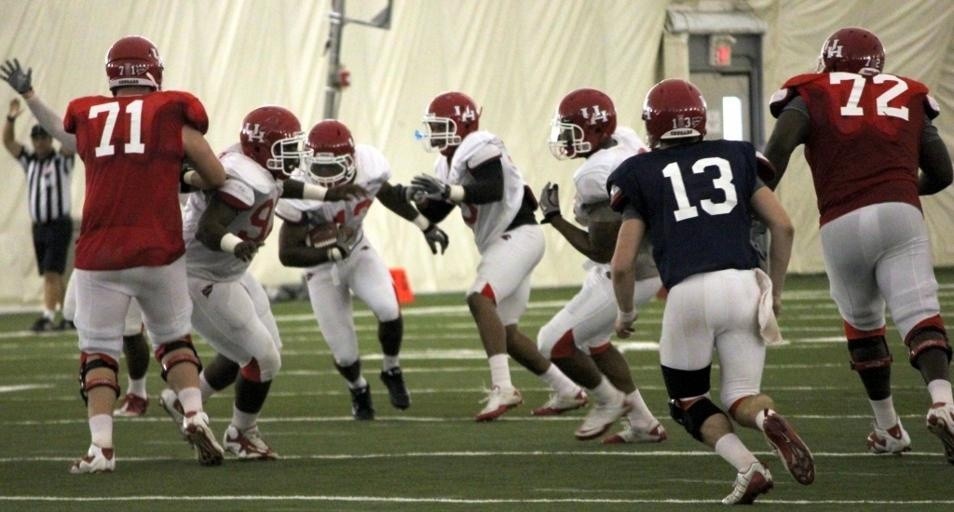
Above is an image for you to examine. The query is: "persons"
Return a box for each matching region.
[539,89,667,441]
[407,92,589,420]
[279,120,450,422]
[0,98,75,332]
[763,28,954,467]
[60,36,226,473]
[1,58,151,418]
[184,106,370,461]
[606,79,817,503]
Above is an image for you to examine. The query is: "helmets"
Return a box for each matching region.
[238,105,315,182]
[104,36,164,96]
[641,78,708,151]
[420,90,483,155]
[305,118,358,189]
[815,27,886,75]
[547,88,618,161]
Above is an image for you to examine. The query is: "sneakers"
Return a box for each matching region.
[573,391,634,439]
[67,443,117,474]
[721,459,774,505]
[31,316,53,332]
[761,407,816,486]
[53,318,76,332]
[379,364,412,411]
[113,392,150,418]
[158,387,185,436]
[348,381,375,421]
[182,410,225,466]
[600,420,668,444]
[925,402,954,463]
[530,389,589,416]
[865,415,912,457]
[474,384,523,422]
[222,424,279,459]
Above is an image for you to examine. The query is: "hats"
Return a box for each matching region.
[30,124,48,136]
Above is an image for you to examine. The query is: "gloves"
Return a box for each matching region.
[410,171,451,200]
[421,221,450,255]
[538,181,561,225]
[614,306,639,339]
[0,58,34,95]
[331,223,365,262]
